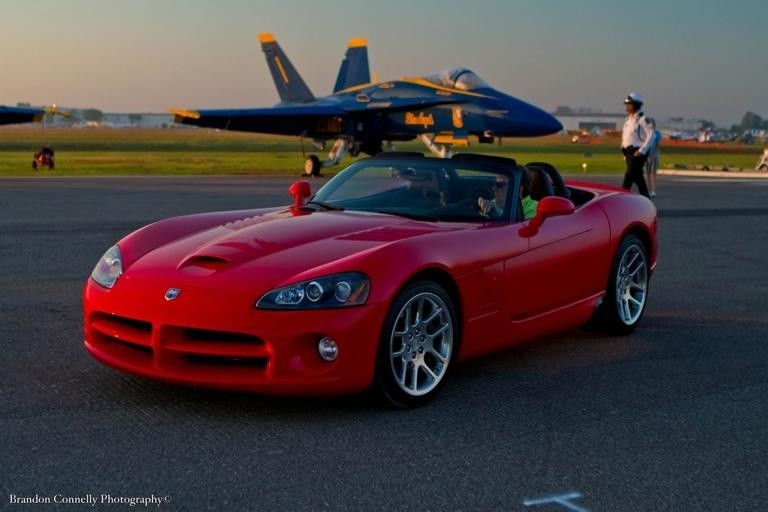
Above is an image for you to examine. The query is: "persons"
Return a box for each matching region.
[620,92,656,199]
[477,165,538,219]
[646,118,663,197]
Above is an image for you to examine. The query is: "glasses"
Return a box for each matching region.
[492,179,508,189]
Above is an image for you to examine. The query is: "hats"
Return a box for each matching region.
[623,92,646,105]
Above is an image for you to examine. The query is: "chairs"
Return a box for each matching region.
[406,162,571,206]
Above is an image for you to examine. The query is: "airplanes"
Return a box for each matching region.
[0,106,45,128]
[167,32,564,177]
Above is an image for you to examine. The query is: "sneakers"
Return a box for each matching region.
[649,191,656,197]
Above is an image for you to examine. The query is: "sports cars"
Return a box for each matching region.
[84,150,659,409]
